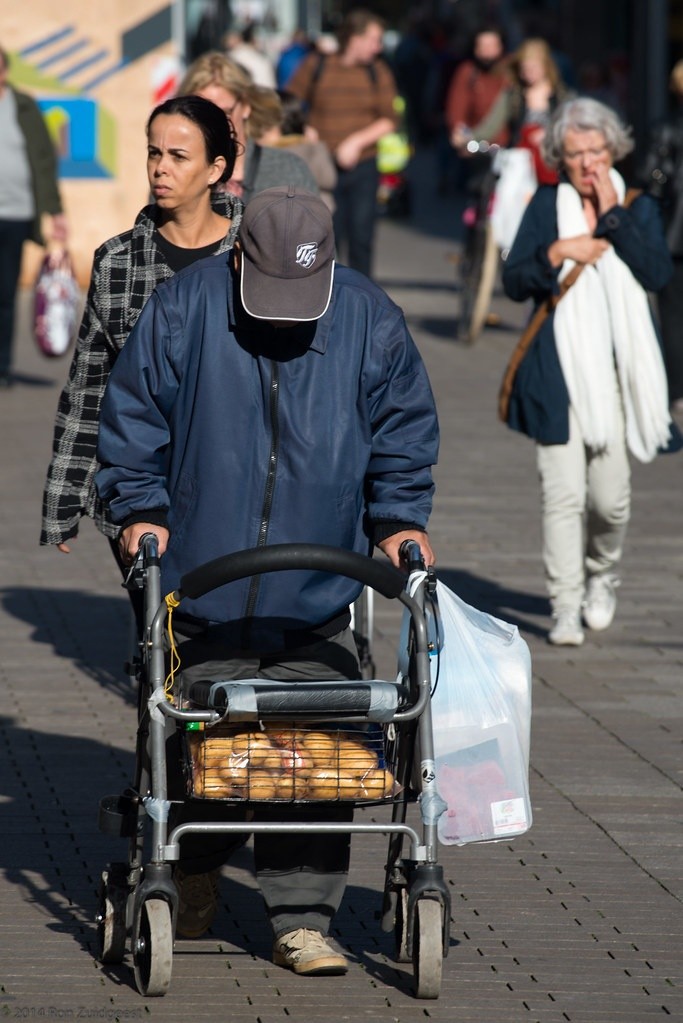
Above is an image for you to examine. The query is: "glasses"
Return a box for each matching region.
[222,97,240,116]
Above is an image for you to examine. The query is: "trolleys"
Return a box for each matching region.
[93,532,460,1001]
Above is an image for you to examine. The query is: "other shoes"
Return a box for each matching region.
[273,929,348,973]
[548,604,584,644]
[172,863,221,939]
[584,572,625,631]
[657,419,683,454]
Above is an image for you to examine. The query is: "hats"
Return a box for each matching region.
[236,185,335,321]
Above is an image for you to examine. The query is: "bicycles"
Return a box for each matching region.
[458,140,531,344]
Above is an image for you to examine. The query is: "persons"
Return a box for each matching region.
[445,20,523,205]
[94,188,441,975]
[37,96,243,639]
[264,91,338,216]
[293,13,404,282]
[631,60,682,453]
[177,0,343,210]
[500,97,675,644]
[0,48,62,386]
[511,38,576,185]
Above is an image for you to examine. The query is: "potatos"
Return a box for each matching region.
[192,731,394,799]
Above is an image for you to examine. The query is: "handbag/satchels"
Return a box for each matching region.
[31,237,83,360]
[497,338,526,422]
[396,569,532,845]
